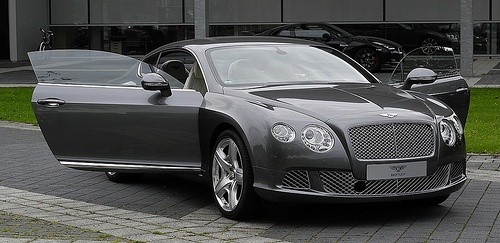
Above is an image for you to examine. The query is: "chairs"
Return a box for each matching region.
[159,58,264,98]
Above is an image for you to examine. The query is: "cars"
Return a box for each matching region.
[26,34,471,220]
[359,24,460,56]
[257,23,407,73]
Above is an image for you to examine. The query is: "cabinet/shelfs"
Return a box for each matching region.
[110,40,122,55]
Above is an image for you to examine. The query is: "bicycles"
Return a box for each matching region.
[38,27,56,51]
